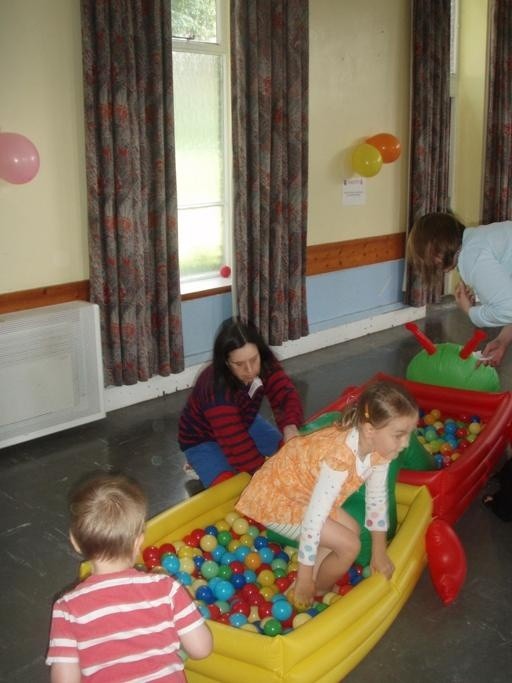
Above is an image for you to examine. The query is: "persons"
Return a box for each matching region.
[176,315,304,487]
[409,208,512,521]
[41,470,214,682]
[232,378,420,613]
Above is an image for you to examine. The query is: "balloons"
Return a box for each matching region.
[0,132,40,185]
[425,517,468,609]
[352,142,382,177]
[366,132,401,163]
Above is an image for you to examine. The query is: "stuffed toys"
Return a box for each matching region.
[404,321,502,394]
